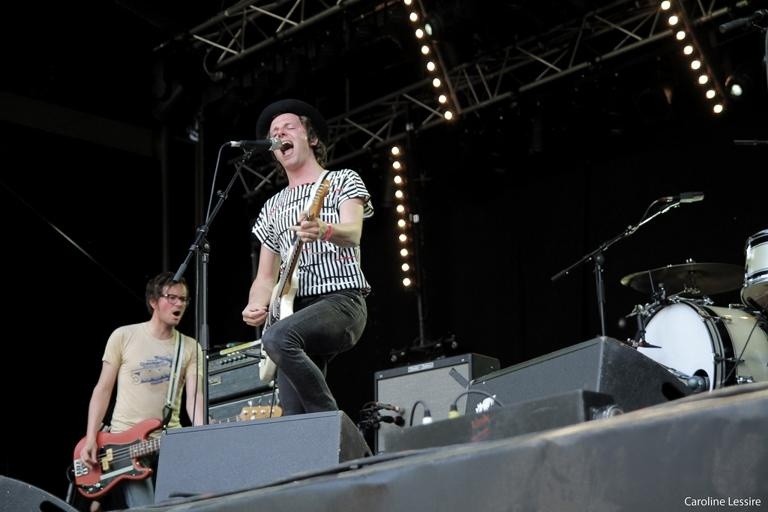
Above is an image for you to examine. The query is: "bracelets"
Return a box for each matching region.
[320,224,333,243]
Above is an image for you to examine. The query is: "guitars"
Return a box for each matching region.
[260,178,330,386]
[73,405,283,497]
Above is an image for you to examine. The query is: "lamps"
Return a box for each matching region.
[401,0,457,123]
[390,140,420,292]
[657,0,743,119]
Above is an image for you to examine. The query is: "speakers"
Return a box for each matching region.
[0,475,80,512]
[465,336,694,414]
[155,411,374,505]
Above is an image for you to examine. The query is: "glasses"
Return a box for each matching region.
[151,293,189,308]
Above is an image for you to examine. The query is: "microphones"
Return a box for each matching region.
[662,192,704,203]
[373,402,405,413]
[230,137,282,149]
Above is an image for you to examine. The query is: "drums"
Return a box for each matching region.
[634,302,768,391]
[740,230,768,308]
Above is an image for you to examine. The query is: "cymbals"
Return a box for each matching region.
[621,262,745,295]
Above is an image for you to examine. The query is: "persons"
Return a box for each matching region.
[79,272,206,510]
[242,111,374,413]
